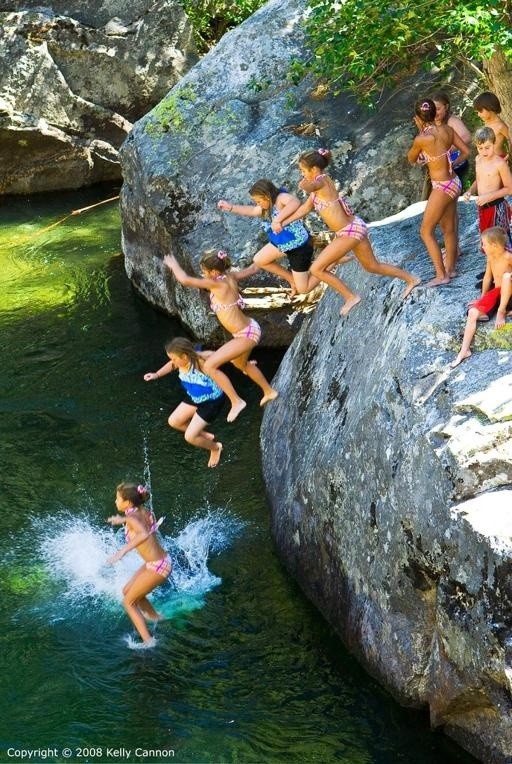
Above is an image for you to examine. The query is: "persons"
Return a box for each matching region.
[407,98,470,287]
[451,227,512,367]
[217,179,355,297]
[281,147,421,316]
[473,92,512,165]
[107,482,172,644]
[144,337,258,469]
[464,127,512,254]
[163,250,278,423]
[433,92,473,266]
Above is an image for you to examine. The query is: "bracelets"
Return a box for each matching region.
[229,205,233,212]
[157,374,159,379]
